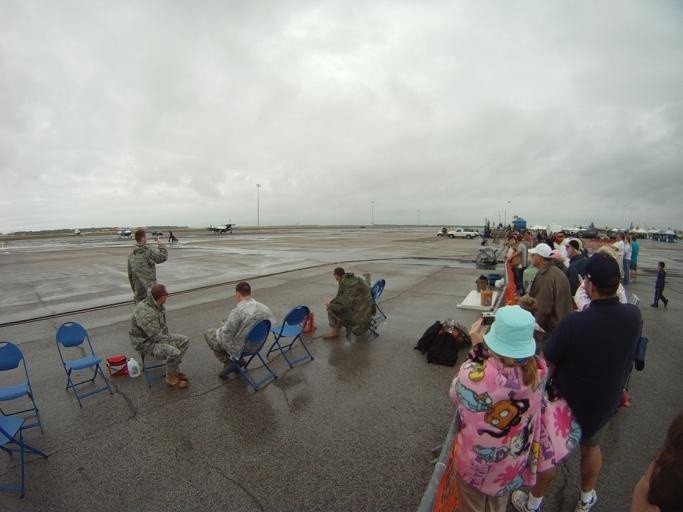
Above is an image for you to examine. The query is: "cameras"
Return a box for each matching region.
[482,312,495,325]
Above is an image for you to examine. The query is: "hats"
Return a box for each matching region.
[483,305,537,359]
[528,242,553,259]
[568,240,579,251]
[574,252,621,288]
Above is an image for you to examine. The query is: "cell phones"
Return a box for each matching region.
[155,236,159,240]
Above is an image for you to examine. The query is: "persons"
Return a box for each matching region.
[128,284,190,389]
[168,231,173,243]
[203,282,276,378]
[323,267,374,339]
[651,262,668,308]
[128,230,168,305]
[451,227,642,512]
[631,412,683,512]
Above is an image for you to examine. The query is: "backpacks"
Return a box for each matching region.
[415,317,475,368]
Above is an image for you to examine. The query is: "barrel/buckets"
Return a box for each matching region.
[476,278,488,292]
[107,356,130,376]
[480,288,493,305]
[488,273,502,287]
[300,313,314,332]
[128,356,140,378]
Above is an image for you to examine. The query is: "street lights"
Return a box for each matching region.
[370,200,374,224]
[505,200,512,226]
[256,183,262,233]
[417,209,420,225]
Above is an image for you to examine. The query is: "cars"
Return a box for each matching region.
[152,231,164,237]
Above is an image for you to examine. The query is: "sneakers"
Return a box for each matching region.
[511,489,541,512]
[575,489,597,512]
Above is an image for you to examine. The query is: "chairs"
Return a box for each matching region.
[0,339,45,435]
[140,351,168,388]
[55,321,113,409]
[265,304,314,369]
[341,285,382,338]
[0,413,47,500]
[223,318,278,392]
[370,278,388,319]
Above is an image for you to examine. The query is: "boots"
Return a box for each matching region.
[165,368,189,388]
[218,358,247,378]
[322,325,341,340]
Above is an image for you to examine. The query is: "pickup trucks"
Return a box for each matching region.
[448,228,480,239]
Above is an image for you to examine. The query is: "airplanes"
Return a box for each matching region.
[206,217,236,237]
[564,222,683,243]
[117,229,134,240]
